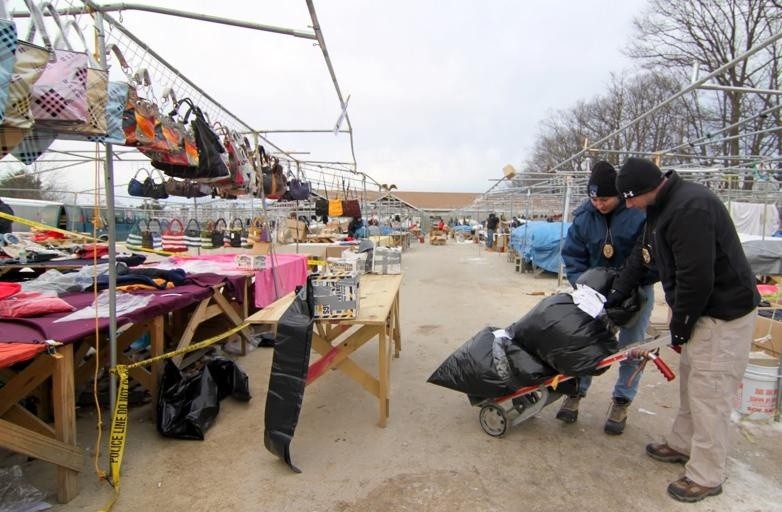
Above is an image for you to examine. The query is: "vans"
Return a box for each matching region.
[40,204,145,243]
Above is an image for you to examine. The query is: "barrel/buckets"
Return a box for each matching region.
[734,351,782,416]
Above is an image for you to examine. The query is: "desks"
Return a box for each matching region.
[244,272,403,429]
[1,250,308,506]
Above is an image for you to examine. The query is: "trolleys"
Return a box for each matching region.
[467,333,686,439]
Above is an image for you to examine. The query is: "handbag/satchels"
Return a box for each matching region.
[1,1,141,165]
[90,268,187,292]
[0,230,110,263]
[314,194,362,219]
[124,214,341,251]
[128,65,312,200]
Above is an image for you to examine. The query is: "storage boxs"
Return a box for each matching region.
[341,245,373,275]
[308,271,361,321]
[373,246,402,275]
[750,306,782,355]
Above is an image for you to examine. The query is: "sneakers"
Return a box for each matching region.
[556,395,581,422]
[604,398,632,435]
[646,442,690,463]
[667,476,723,501]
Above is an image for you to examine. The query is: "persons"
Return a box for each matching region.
[606,157,762,503]
[347,212,553,248]
[554,161,655,436]
[1,201,15,235]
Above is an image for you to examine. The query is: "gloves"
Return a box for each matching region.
[603,287,626,309]
[669,329,691,346]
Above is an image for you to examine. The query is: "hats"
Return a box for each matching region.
[614,157,665,198]
[586,161,619,199]
